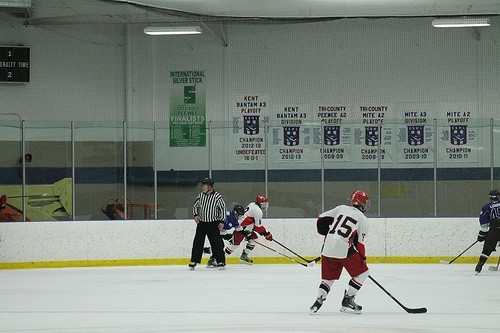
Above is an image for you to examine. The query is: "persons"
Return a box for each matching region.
[309,190,370,315]
[188,177,226,270]
[203,205,244,268]
[224,195,272,264]
[473,190,500,276]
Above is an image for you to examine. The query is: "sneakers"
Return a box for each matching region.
[189,262,196,270]
[202,248,205,256]
[310,295,326,315]
[217,262,226,270]
[206,257,217,268]
[340,289,362,313]
[475,261,484,275]
[240,249,253,265]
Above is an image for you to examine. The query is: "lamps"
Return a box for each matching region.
[432,18,491,27]
[143,25,202,35]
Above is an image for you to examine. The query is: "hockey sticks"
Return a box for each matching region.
[489,253,500,272]
[368,274,427,314]
[252,239,315,268]
[273,238,322,264]
[439,240,479,265]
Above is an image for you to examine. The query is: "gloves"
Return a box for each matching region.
[361,255,369,270]
[477,230,489,242]
[264,231,272,241]
[225,234,236,245]
[245,230,257,239]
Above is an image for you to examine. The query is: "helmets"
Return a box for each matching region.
[351,191,369,212]
[232,205,245,220]
[202,178,215,186]
[489,190,500,201]
[256,195,269,211]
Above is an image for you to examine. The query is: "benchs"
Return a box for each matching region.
[107,202,160,220]
